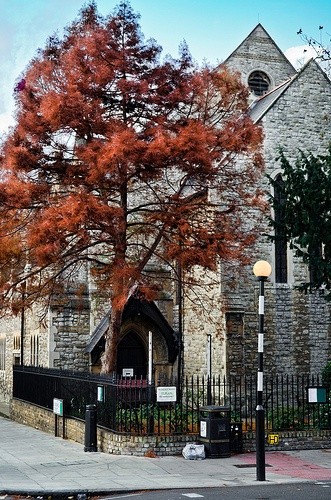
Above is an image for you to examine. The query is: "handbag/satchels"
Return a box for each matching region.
[182,444,206,460]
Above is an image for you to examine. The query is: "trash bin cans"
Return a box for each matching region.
[197,406,231,459]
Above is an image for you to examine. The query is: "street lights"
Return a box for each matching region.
[253,260,271,482]
